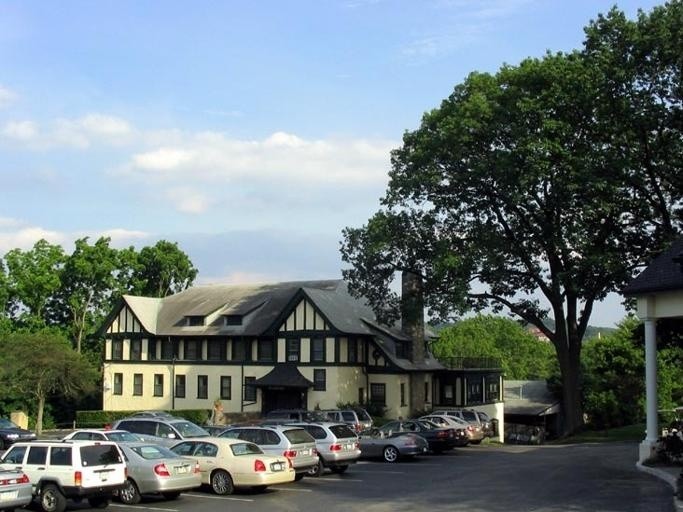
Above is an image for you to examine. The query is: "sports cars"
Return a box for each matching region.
[354,427,429,462]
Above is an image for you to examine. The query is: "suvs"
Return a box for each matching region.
[113,418,212,447]
[312,410,374,432]
[432,410,494,444]
[216,425,319,482]
[282,422,362,477]
[0,440,128,512]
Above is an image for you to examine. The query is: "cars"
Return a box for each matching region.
[0,418,37,450]
[417,415,484,447]
[168,438,296,495]
[380,420,456,453]
[0,465,33,509]
[61,428,143,442]
[113,442,202,505]
